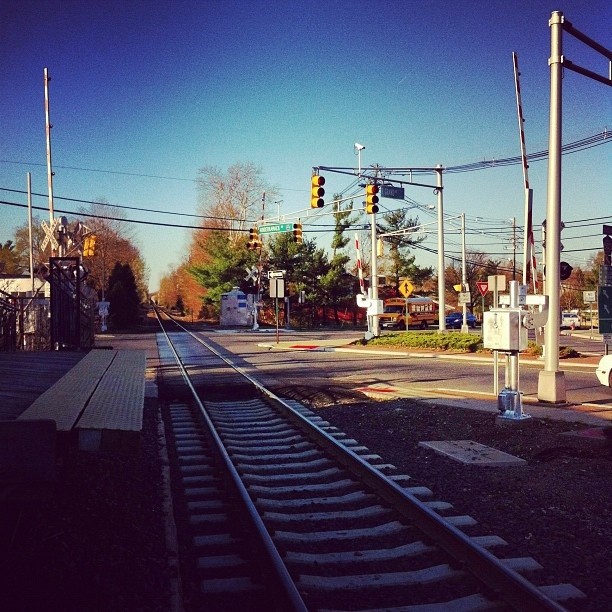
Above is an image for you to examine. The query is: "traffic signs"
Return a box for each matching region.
[268,270,284,278]
[258,223,296,234]
[598,285,612,335]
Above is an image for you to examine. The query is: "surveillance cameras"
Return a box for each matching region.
[354,143,366,151]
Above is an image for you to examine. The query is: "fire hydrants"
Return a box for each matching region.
[571,320,575,331]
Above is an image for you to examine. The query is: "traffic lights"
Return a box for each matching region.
[248,229,262,250]
[453,284,464,292]
[294,224,302,247]
[377,239,384,257]
[84,232,98,259]
[366,184,379,214]
[312,172,325,210]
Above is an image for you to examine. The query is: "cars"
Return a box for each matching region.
[563,313,583,322]
[447,313,476,328]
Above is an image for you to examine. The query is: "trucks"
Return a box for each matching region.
[380,298,435,330]
